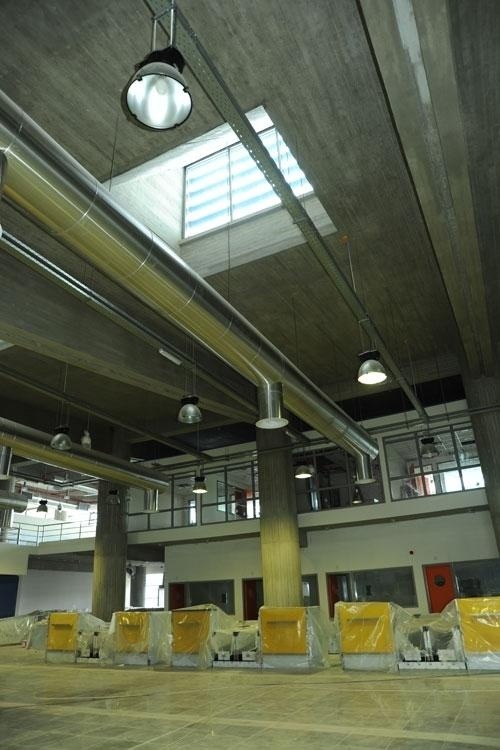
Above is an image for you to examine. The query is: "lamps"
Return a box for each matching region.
[49,400,72,451]
[352,487,363,504]
[293,440,313,479]
[352,316,442,461]
[176,336,209,496]
[123,0,194,134]
[35,483,48,513]
[105,484,122,506]
